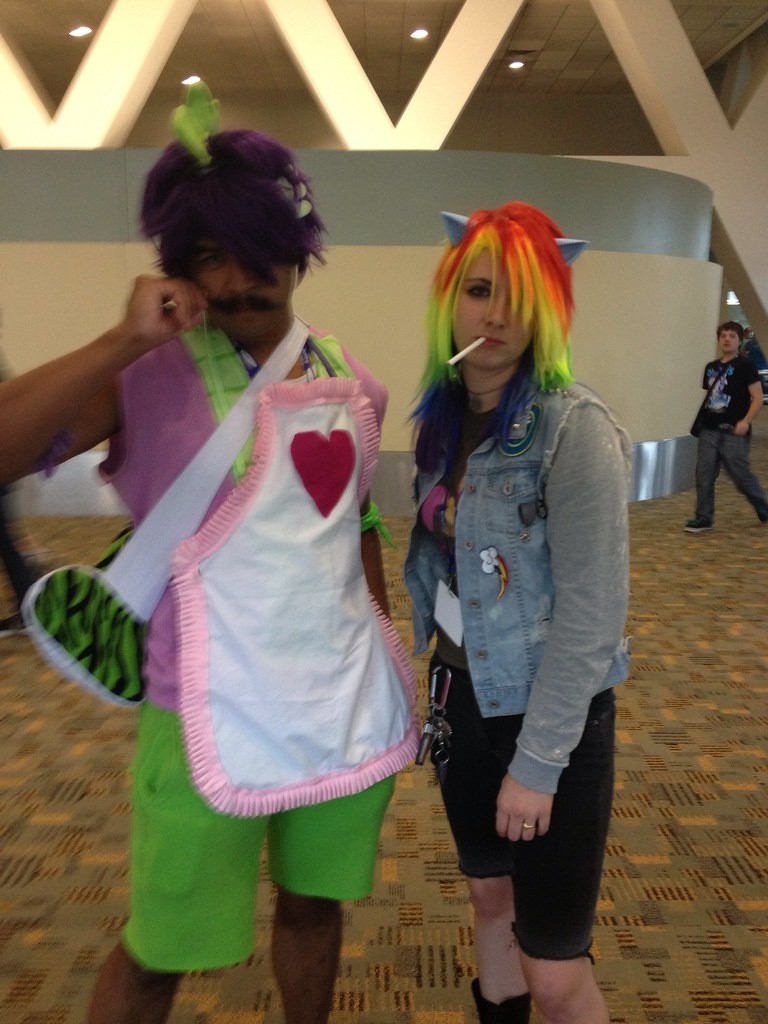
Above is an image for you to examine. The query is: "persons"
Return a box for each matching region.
[0,83,420,1024]
[0,352,47,636]
[684,320,768,533]
[404,202,631,1024]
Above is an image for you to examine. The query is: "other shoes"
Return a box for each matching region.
[0,614,27,637]
[684,518,713,532]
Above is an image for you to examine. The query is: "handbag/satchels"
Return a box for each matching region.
[690,403,708,437]
[20,526,151,706]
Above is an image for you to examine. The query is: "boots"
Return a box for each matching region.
[471,979,532,1024]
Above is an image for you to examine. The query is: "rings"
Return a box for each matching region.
[523,823,535,829]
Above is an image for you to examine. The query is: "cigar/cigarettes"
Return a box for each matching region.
[448,337,486,366]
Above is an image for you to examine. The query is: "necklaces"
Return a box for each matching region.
[466,383,508,410]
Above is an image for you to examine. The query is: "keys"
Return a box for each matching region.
[425,704,453,787]
